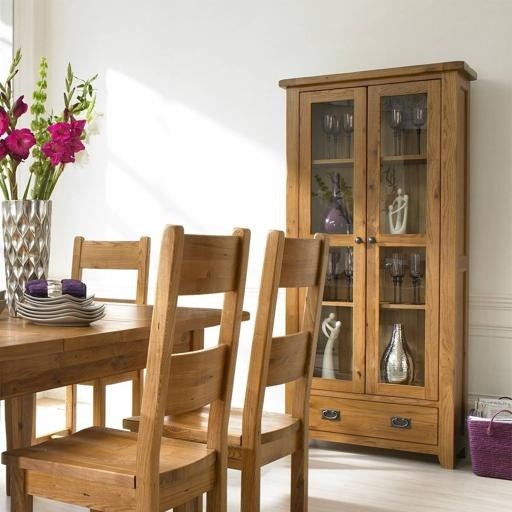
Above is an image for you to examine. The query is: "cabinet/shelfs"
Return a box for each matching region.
[277,59,478,470]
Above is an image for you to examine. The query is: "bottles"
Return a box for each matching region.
[319,172,352,234]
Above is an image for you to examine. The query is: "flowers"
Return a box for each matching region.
[0,47,102,199]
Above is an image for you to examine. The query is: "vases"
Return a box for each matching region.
[1,200,54,318]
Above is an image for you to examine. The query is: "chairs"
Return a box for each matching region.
[64,233,151,437]
[123,231,328,510]
[1,225,254,512]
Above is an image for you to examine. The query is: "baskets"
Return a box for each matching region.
[466,397,512,480]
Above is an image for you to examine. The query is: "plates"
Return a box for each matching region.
[16,293,106,327]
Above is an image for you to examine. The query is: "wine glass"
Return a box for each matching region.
[321,113,341,159]
[411,108,426,154]
[386,108,406,156]
[387,252,426,304]
[342,114,354,160]
[324,247,353,303]
